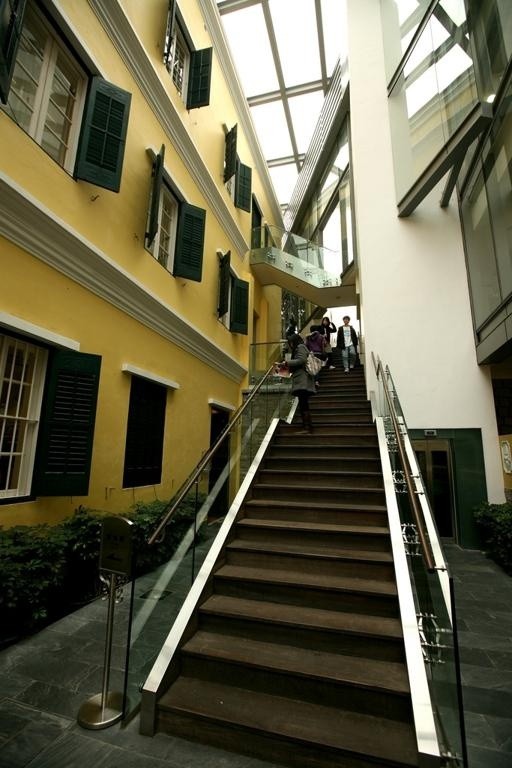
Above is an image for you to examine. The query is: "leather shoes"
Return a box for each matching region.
[294,428,312,434]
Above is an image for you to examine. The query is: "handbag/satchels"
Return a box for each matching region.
[302,345,323,377]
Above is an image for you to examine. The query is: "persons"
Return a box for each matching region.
[305,325,327,388]
[336,316,358,373]
[318,316,337,371]
[282,333,318,436]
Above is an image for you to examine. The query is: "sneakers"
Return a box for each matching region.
[315,380,320,389]
[344,367,350,372]
[329,365,336,370]
[349,363,355,369]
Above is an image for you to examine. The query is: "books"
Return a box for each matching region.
[272,361,293,379]
